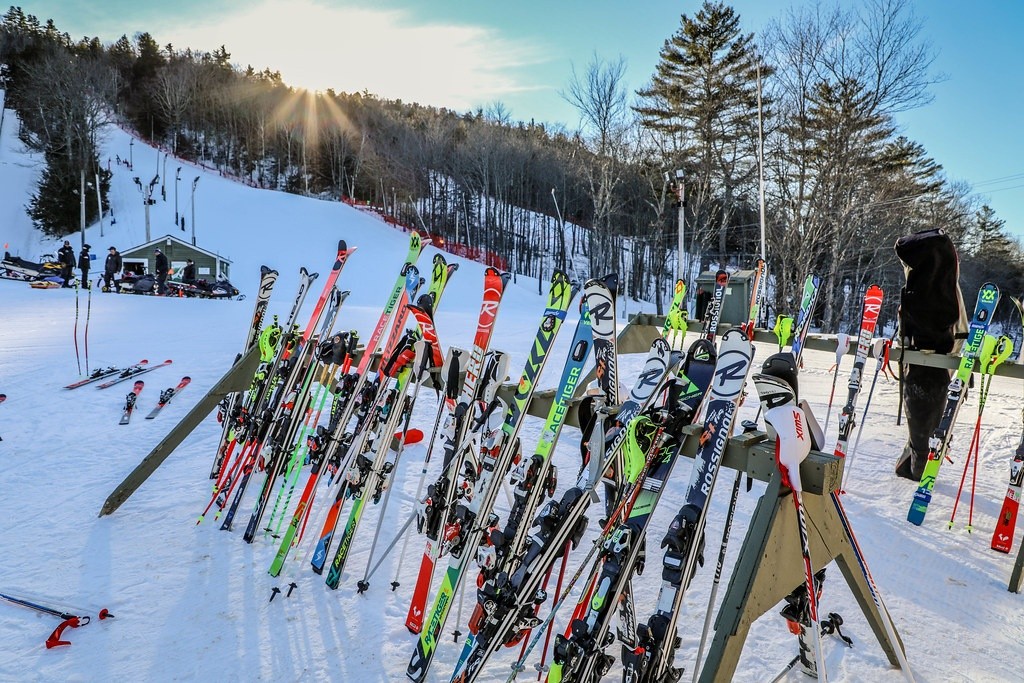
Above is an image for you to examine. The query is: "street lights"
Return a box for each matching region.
[131,173,160,242]
[664,167,689,322]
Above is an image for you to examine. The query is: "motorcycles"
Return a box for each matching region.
[0,251,75,282]
[166,271,245,300]
[103,274,161,297]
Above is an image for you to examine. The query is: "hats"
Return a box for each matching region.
[154,247,161,254]
[64,240,69,245]
[108,247,116,250]
[84,244,91,248]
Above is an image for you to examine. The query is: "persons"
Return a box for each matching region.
[102,246,122,293]
[182,259,195,298]
[78,244,91,289]
[154,247,168,296]
[58,241,76,288]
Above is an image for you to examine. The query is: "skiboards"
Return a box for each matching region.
[120,376,191,424]
[63,359,171,393]
[198,232,909,683]
[655,269,1024,593]
